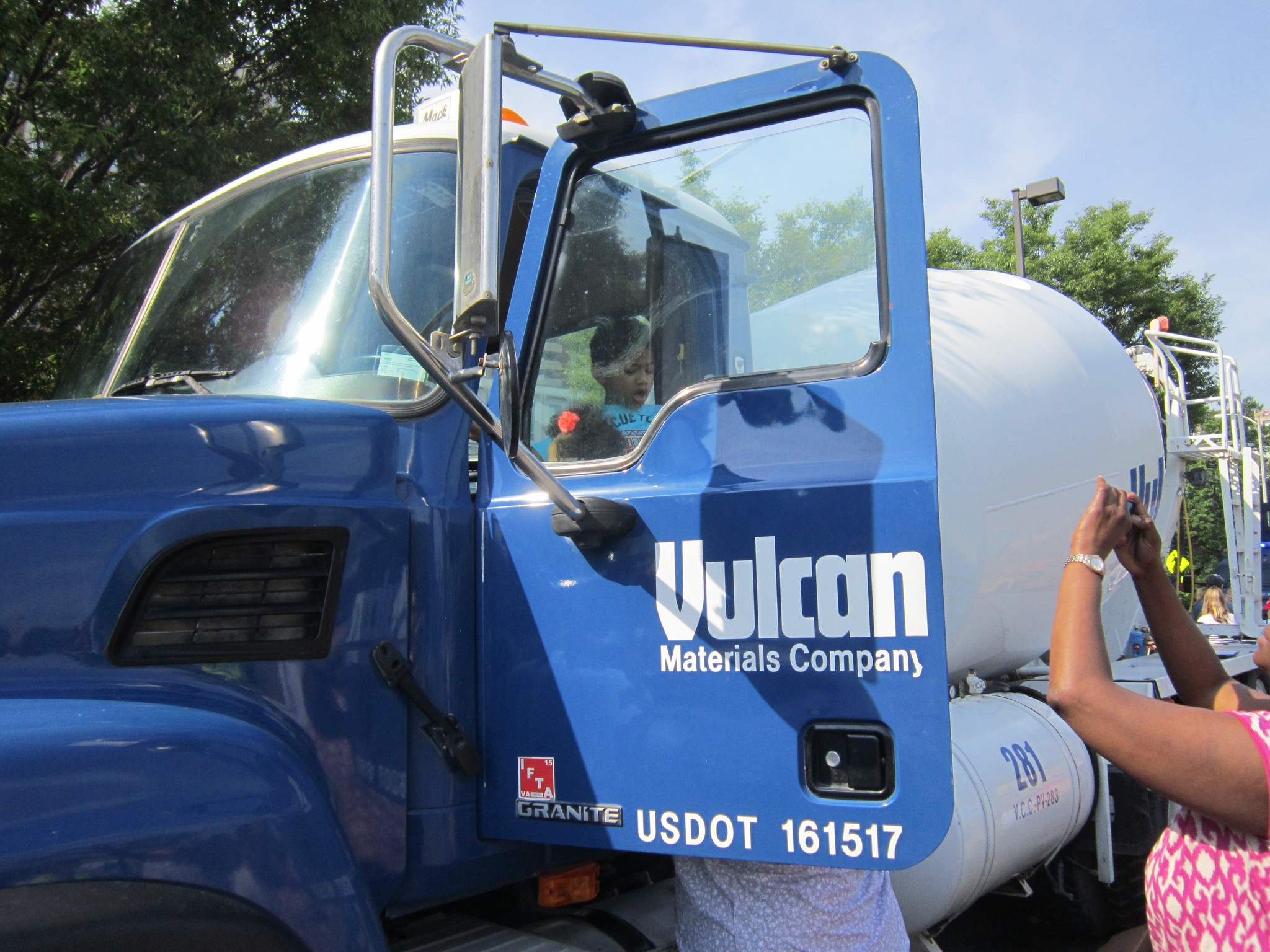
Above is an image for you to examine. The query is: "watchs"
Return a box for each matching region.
[1064,553,1106,579]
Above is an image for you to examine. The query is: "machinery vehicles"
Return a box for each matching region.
[3,21,1270,952]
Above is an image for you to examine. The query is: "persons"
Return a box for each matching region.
[674,855,913,952]
[544,401,627,465]
[469,315,671,455]
[1185,572,1270,645]
[1045,476,1270,952]
